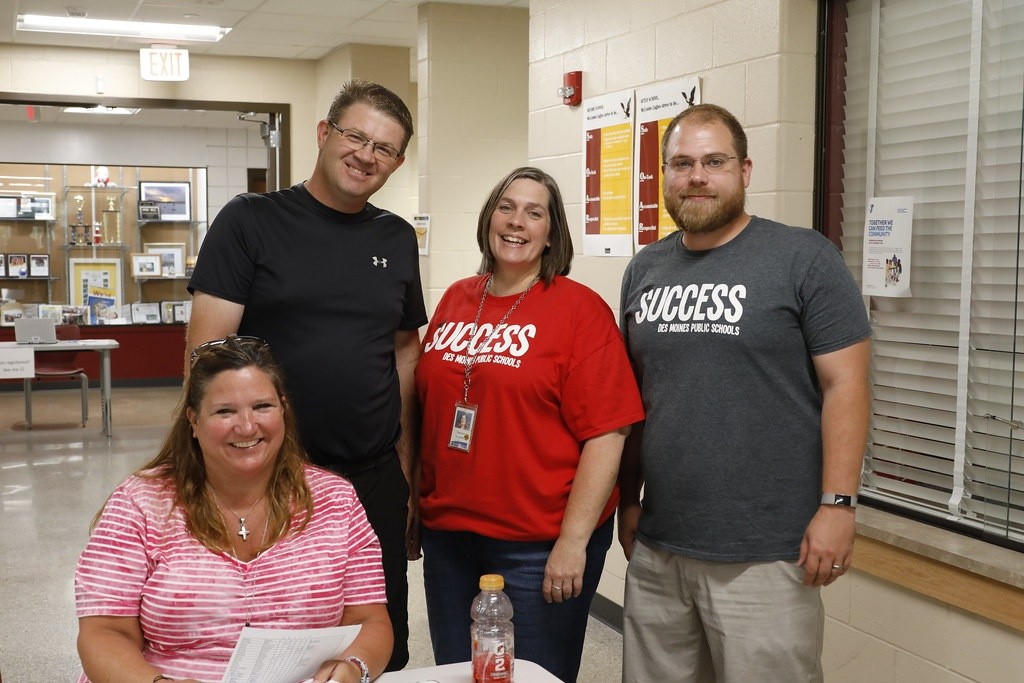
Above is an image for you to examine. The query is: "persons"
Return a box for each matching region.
[617,104,873,683]
[74,336,394,683]
[885,254,902,282]
[184,79,428,673]
[457,414,469,430]
[405,165,646,683]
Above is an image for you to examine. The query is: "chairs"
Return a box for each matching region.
[25,325,90,429]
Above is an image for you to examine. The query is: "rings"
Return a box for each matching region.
[832,565,844,570]
[552,585,562,590]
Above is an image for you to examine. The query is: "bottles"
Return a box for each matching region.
[470,574,515,683]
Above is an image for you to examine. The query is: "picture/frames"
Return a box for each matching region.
[0,181,191,324]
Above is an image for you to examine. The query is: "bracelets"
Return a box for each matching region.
[344,656,370,683]
[821,493,857,508]
[153,675,174,683]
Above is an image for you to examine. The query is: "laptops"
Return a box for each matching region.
[14,318,59,344]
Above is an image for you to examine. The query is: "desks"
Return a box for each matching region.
[0,339,119,437]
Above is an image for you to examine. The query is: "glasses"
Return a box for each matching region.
[189,335,271,380]
[664,155,739,173]
[328,119,400,164]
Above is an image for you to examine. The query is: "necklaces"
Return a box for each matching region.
[202,472,274,629]
